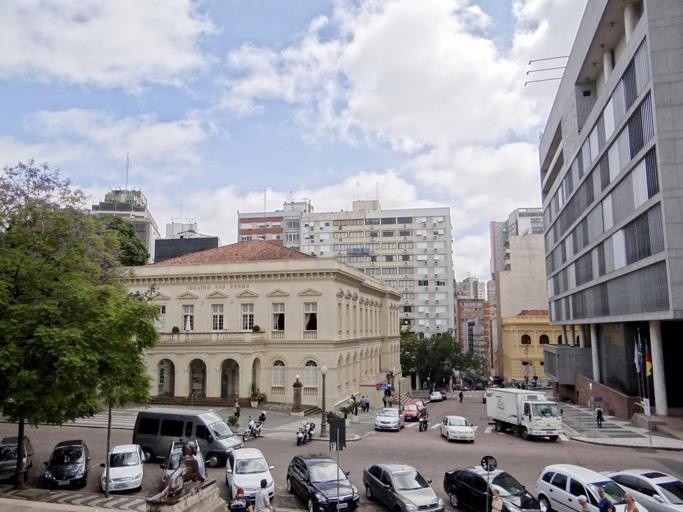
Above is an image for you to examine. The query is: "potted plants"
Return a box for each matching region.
[337,404,353,427]
[351,399,366,424]
[248,382,265,410]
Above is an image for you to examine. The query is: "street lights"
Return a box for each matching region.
[396,377,402,412]
[318,365,328,437]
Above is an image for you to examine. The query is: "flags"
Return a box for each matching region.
[637,330,643,364]
[634,340,641,373]
[645,341,652,376]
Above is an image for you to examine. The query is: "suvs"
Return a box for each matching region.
[0,435,33,482]
[158,438,209,492]
[224,446,276,505]
[284,452,359,512]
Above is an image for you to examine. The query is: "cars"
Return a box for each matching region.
[413,399,424,411]
[597,467,682,512]
[441,464,544,512]
[362,463,445,512]
[41,438,92,490]
[455,375,502,405]
[373,407,406,432]
[429,391,442,402]
[400,402,420,422]
[96,443,146,493]
[439,415,476,444]
[440,391,447,400]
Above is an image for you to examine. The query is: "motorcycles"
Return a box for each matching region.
[417,414,429,432]
[294,420,315,448]
[242,416,266,443]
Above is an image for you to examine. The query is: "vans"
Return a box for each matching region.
[533,461,650,512]
[130,408,243,469]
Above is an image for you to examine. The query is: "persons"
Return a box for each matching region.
[623,492,639,512]
[305,422,310,435]
[595,405,603,428]
[417,407,428,427]
[227,488,254,512]
[383,394,394,408]
[254,479,273,512]
[348,393,370,412]
[491,489,503,512]
[578,495,593,512]
[234,398,241,417]
[459,391,463,403]
[598,488,613,512]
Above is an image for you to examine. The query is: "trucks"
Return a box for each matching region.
[484,387,564,443]
